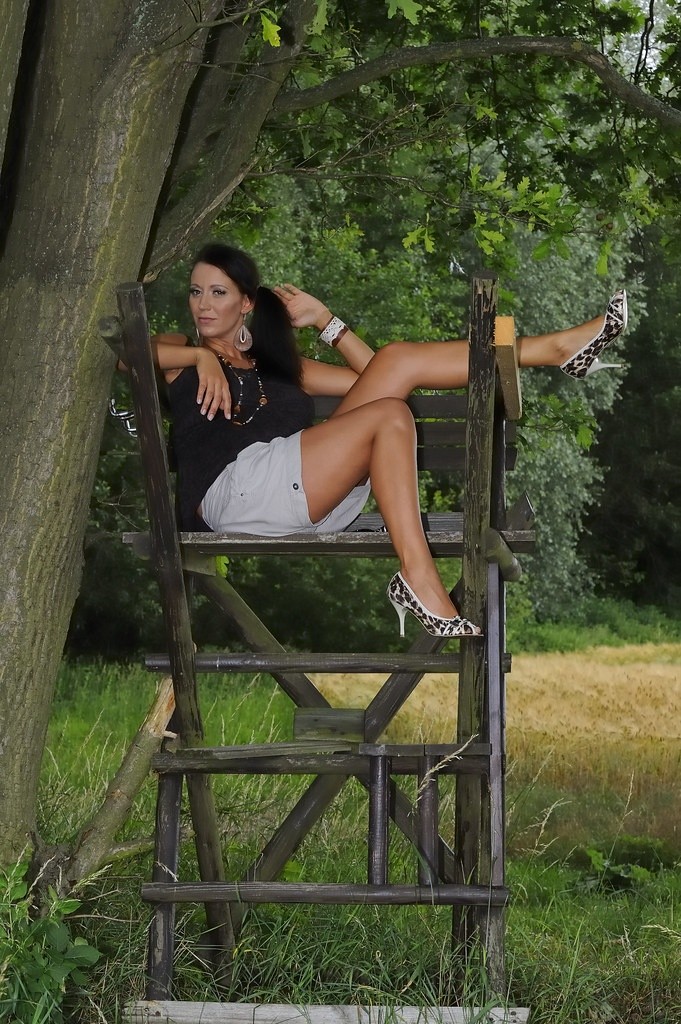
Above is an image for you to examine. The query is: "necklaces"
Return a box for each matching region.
[210,348,267,425]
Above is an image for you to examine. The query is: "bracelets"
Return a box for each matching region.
[318,316,349,347]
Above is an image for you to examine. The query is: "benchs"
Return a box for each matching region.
[107,283,533,1024]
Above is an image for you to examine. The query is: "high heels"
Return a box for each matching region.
[559,289,628,380]
[386,570,484,638]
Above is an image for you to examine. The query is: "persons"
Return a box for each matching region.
[118,243,628,640]
